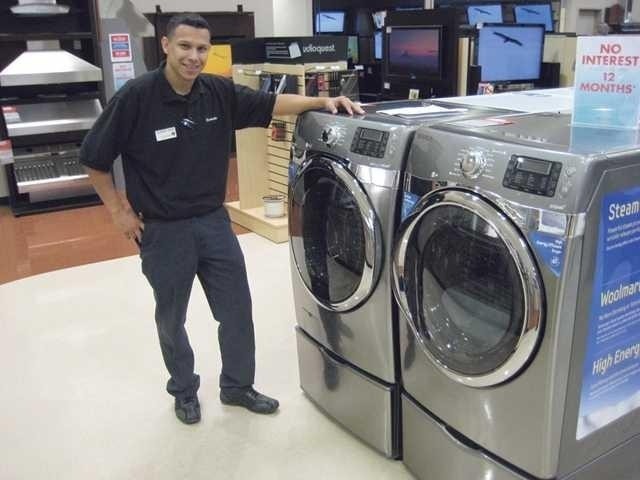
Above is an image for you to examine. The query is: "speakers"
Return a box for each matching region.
[534,62,560,88]
[466,65,481,96]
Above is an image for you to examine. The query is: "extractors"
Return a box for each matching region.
[0,41,107,100]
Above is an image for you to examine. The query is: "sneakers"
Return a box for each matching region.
[220,385,279,414]
[175,392,201,424]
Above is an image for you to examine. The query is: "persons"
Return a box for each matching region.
[78,14,366,424]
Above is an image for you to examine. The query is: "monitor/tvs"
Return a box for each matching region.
[464,2,505,27]
[473,22,546,85]
[512,2,555,33]
[314,7,424,65]
[385,25,444,81]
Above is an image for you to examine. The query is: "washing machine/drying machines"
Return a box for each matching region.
[288,88,640,480]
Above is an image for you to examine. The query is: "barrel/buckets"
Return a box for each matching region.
[262,193,286,217]
